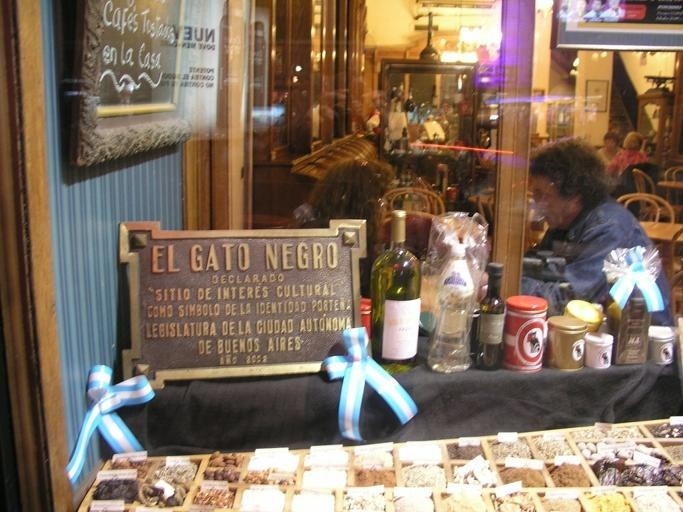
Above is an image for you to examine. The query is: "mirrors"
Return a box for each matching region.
[377,58,478,196]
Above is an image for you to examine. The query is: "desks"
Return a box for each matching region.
[102,337,680,461]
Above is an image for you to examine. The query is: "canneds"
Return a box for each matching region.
[505,294,615,373]
[648,324,674,365]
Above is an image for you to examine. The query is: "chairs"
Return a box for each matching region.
[379,187,446,214]
[615,163,683,320]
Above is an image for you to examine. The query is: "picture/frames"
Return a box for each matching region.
[68,0,194,171]
[114,219,370,391]
[549,0,683,54]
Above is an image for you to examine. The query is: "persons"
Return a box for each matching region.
[280,157,441,299]
[594,132,623,169]
[555,1,627,24]
[606,132,649,180]
[478,135,671,329]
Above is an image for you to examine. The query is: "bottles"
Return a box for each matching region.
[370,210,422,375]
[501,294,677,373]
[478,261,507,371]
[427,247,474,373]
